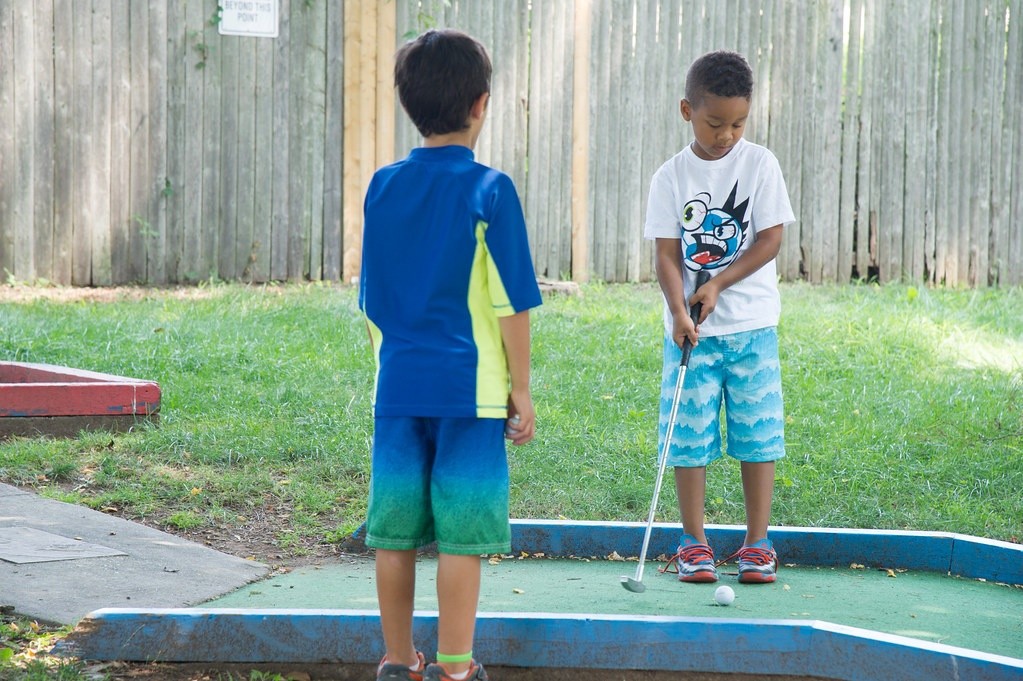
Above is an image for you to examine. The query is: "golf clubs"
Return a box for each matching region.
[616,265,712,594]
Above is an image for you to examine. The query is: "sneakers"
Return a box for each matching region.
[658,534,719,582]
[422,658,489,681]
[716,538,778,582]
[377,649,426,681]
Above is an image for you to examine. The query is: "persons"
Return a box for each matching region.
[357,28,545,681]
[644,52,797,582]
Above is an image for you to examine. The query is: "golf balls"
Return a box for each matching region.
[713,584,735,606]
